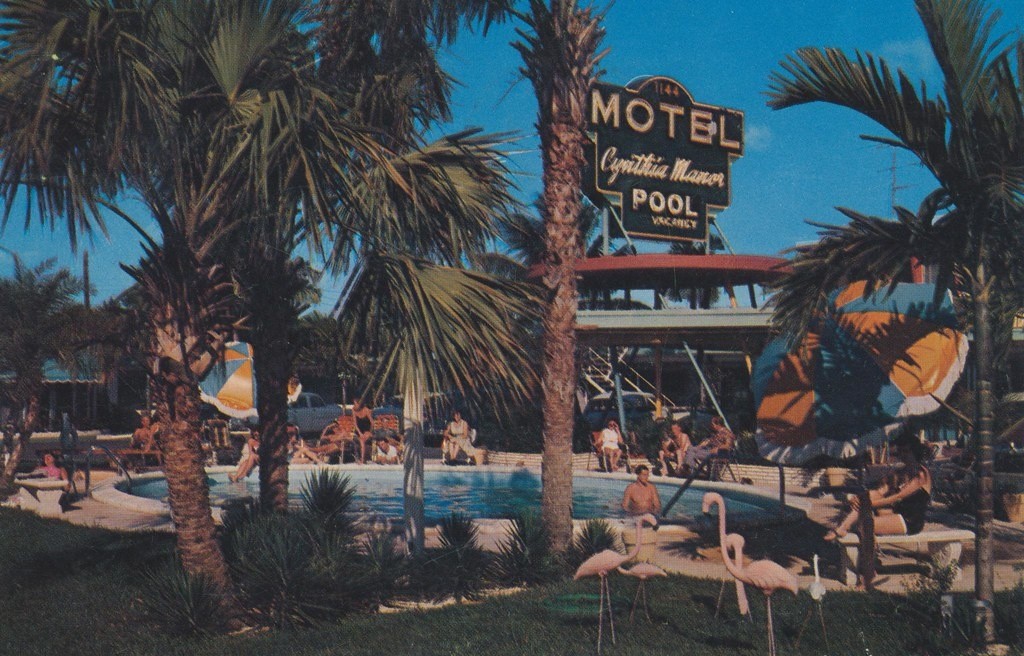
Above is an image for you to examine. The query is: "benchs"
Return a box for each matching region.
[13,478,69,518]
[34,448,163,471]
[833,530,974,587]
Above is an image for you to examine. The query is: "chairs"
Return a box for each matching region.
[286,414,404,466]
[442,429,477,465]
[586,431,738,483]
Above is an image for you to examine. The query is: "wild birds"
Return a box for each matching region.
[573,492,835,656]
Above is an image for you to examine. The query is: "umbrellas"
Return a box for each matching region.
[189,341,302,418]
[590,388,653,400]
[749,277,1017,467]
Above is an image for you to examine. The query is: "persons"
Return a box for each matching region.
[228,428,260,484]
[443,411,467,466]
[592,420,619,469]
[622,464,660,527]
[109,413,164,471]
[287,397,404,467]
[822,433,932,541]
[658,416,734,478]
[32,453,70,492]
